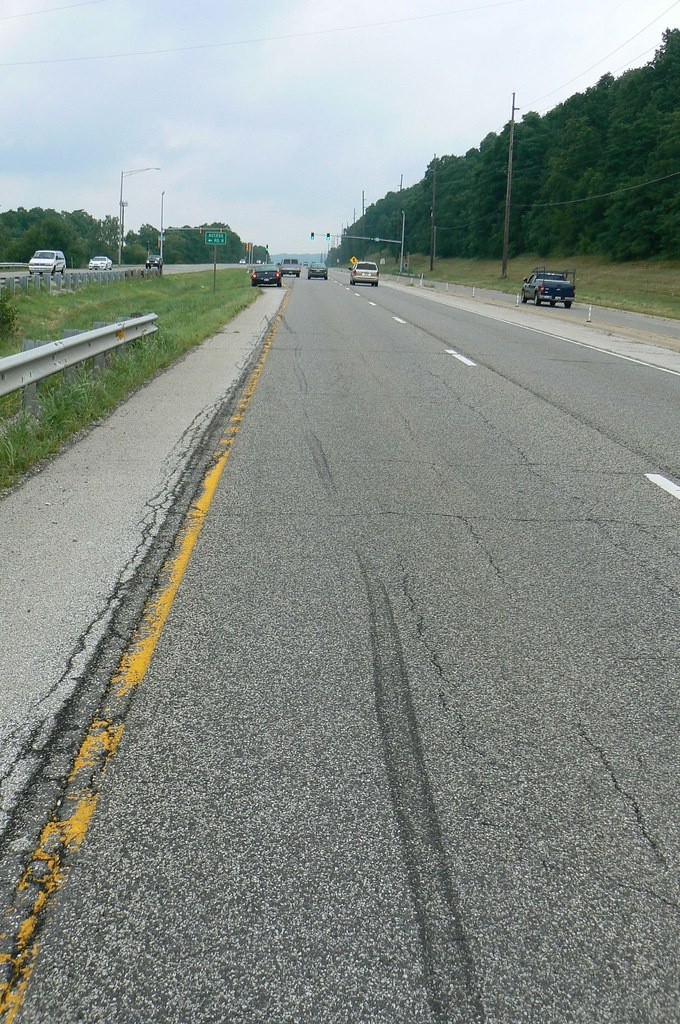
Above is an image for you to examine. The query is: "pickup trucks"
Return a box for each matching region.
[521,266,576,309]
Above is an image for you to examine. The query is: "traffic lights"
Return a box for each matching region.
[310,233,314,240]
[265,248,268,254]
[326,233,330,240]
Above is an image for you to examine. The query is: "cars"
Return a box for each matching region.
[239,259,246,264]
[88,256,112,271]
[306,262,328,280]
[250,264,282,287]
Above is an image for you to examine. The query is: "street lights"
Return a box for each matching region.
[160,190,166,259]
[118,167,161,266]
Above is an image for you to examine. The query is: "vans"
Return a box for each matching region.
[277,258,302,277]
[28,249,67,276]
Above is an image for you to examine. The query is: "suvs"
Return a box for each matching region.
[348,261,379,287]
[145,254,162,269]
